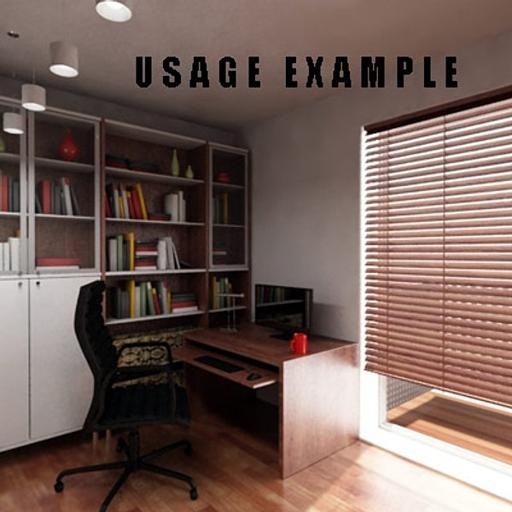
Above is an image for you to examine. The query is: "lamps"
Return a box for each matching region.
[4,112,26,135]
[93,0,132,23]
[50,45,80,78]
[22,85,46,111]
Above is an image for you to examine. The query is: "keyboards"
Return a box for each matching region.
[193,355,245,374]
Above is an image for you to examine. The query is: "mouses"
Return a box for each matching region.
[247,372,262,380]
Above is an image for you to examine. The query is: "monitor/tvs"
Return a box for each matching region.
[255,284,314,341]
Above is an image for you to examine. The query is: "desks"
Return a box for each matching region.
[181,320,361,475]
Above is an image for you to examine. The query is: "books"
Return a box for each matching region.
[150,211,171,221]
[257,286,285,304]
[134,238,160,269]
[0,169,20,212]
[212,191,228,224]
[213,247,227,257]
[164,191,186,222]
[109,279,172,319]
[105,181,149,220]
[35,176,82,216]
[210,276,231,310]
[158,236,196,270]
[0,237,20,272]
[109,232,136,271]
[35,257,82,271]
[171,292,199,313]
[278,314,303,328]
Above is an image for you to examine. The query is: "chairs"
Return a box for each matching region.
[54,280,209,512]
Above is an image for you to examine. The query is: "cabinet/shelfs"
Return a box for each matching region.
[0,274,99,452]
[0,101,101,272]
[111,270,252,382]
[209,142,254,270]
[103,98,209,271]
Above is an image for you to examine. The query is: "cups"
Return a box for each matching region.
[290,333,307,355]
